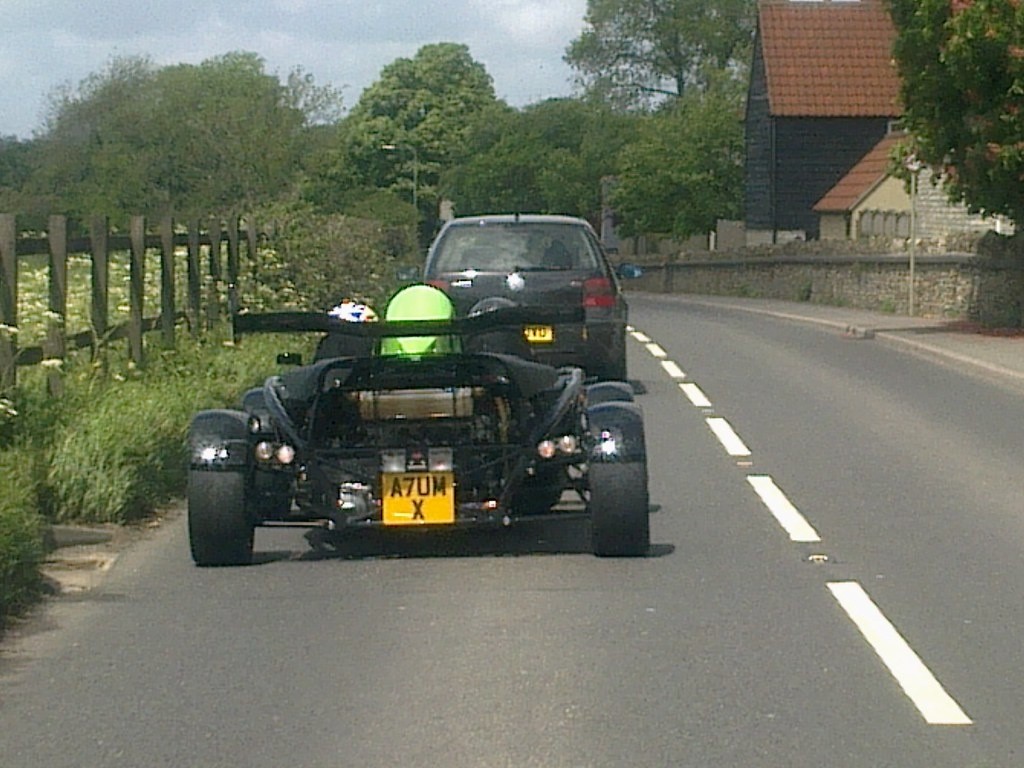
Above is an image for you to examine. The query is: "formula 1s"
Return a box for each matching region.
[186,312,650,567]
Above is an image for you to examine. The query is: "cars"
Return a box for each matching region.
[423,212,628,382]
